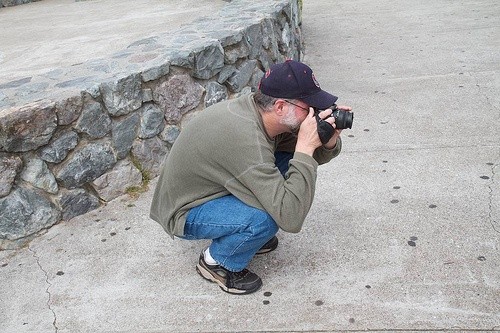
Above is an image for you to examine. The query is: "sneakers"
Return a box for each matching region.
[196,236,279,295]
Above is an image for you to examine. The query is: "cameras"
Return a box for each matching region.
[313,107,354,129]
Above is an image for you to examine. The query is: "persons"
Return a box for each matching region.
[150,60,352,295]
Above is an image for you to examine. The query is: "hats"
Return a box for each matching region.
[258,60,338,110]
